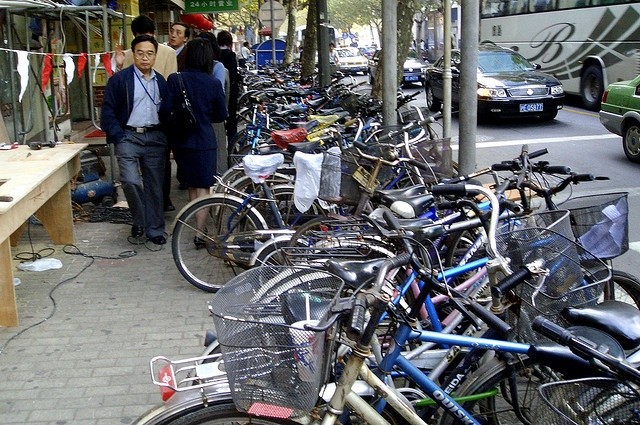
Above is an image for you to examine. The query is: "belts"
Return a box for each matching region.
[125,126,160,133]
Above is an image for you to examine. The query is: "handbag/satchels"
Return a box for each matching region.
[165,72,197,136]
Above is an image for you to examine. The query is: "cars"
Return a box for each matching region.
[335,47,368,76]
[425,46,565,124]
[368,49,433,87]
[599,74,640,163]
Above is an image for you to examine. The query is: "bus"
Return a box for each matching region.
[479,0,640,110]
[295,24,339,65]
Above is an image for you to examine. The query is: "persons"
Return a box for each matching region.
[101,34,166,245]
[199,32,228,175]
[216,31,237,169]
[329,43,339,65]
[115,15,177,211]
[159,39,228,250]
[163,22,190,190]
[238,42,250,68]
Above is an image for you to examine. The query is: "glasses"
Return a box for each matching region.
[134,50,156,59]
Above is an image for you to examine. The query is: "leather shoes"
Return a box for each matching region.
[148,234,166,243]
[131,225,142,238]
[164,205,175,211]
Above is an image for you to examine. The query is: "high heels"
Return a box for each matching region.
[193,235,207,248]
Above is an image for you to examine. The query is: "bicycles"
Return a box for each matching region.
[230,92,443,162]
[237,68,367,92]
[130,150,640,425]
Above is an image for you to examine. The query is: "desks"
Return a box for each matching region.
[0,142,89,327]
